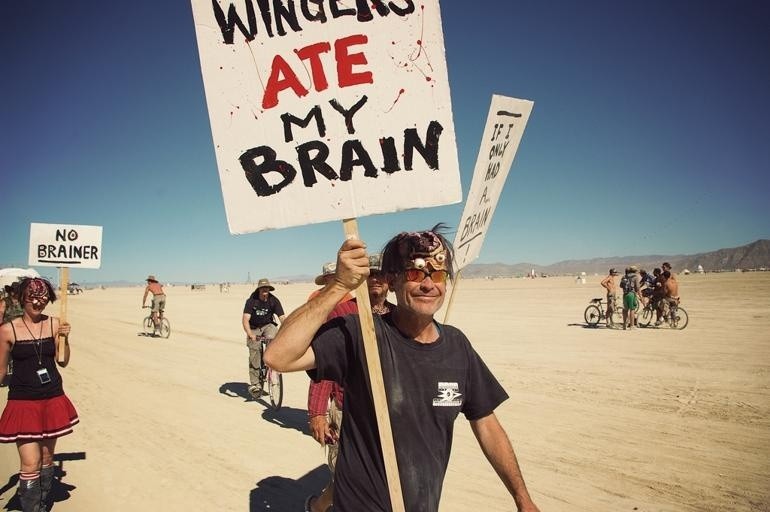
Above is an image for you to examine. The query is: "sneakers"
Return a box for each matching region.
[253,384,260,393]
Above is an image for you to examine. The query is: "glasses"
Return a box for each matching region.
[402,268,447,283]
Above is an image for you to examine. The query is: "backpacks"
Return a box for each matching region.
[621,274,636,294]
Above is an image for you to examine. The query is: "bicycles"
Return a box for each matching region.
[141,303,172,339]
[250,336,284,411]
[584,290,689,329]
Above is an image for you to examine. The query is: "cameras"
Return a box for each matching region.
[37,369,51,384]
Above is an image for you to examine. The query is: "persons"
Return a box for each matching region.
[662,270,680,323]
[637,267,657,297]
[620,265,643,329]
[301,253,397,511]
[261,222,542,512]
[0,276,83,512]
[600,268,619,329]
[304,261,354,307]
[661,263,678,283]
[143,275,167,332]
[650,267,667,323]
[0,282,25,379]
[243,277,287,400]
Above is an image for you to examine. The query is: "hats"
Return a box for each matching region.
[315,263,337,285]
[146,275,158,282]
[255,279,274,292]
[4,282,19,292]
[368,254,383,272]
